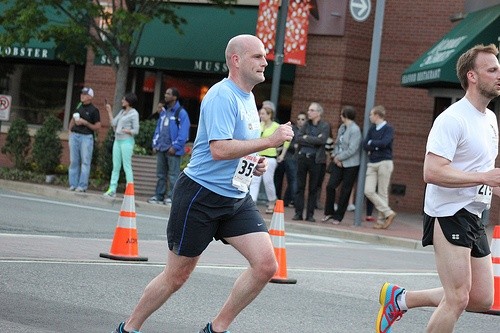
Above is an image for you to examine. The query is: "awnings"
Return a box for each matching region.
[400,4,500,88]
[93,4,296,82]
[0,0,92,65]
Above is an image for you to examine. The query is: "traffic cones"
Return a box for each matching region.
[477,225,500,315]
[265,200,297,284]
[98,181,149,262]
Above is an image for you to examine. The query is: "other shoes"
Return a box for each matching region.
[105,188,116,197]
[69,186,75,191]
[111,321,145,333]
[321,214,333,222]
[305,216,315,222]
[200,322,231,333]
[265,201,274,213]
[291,214,303,220]
[334,203,338,211]
[163,198,172,206]
[383,212,396,228]
[333,220,340,225]
[372,223,384,229]
[348,204,356,211]
[75,187,85,192]
[365,216,374,222]
[147,196,164,204]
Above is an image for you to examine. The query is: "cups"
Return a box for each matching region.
[72,112,80,119]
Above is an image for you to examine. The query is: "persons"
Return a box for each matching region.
[252,100,396,232]
[147,87,191,204]
[148,100,166,121]
[376,43,500,333]
[68,87,102,192]
[103,93,140,199]
[110,34,295,333]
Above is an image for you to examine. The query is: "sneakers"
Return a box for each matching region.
[377,282,405,333]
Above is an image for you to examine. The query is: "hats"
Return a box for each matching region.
[82,87,94,97]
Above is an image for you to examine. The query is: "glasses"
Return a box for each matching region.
[297,118,305,121]
